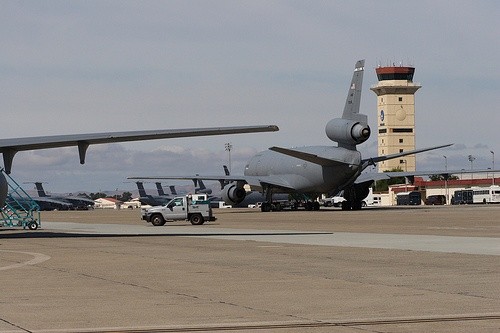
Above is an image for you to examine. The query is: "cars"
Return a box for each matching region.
[319,198,339,208]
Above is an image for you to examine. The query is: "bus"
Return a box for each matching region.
[472,187,500,205]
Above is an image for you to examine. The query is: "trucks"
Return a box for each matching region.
[450,189,472,204]
[331,184,375,206]
[140,196,218,226]
[396,191,421,206]
[425,195,446,206]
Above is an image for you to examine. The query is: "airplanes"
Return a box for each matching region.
[125,57,456,212]
[0,121,278,208]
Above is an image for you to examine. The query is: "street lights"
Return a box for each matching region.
[490,150,495,170]
[443,155,448,169]
[468,154,474,171]
